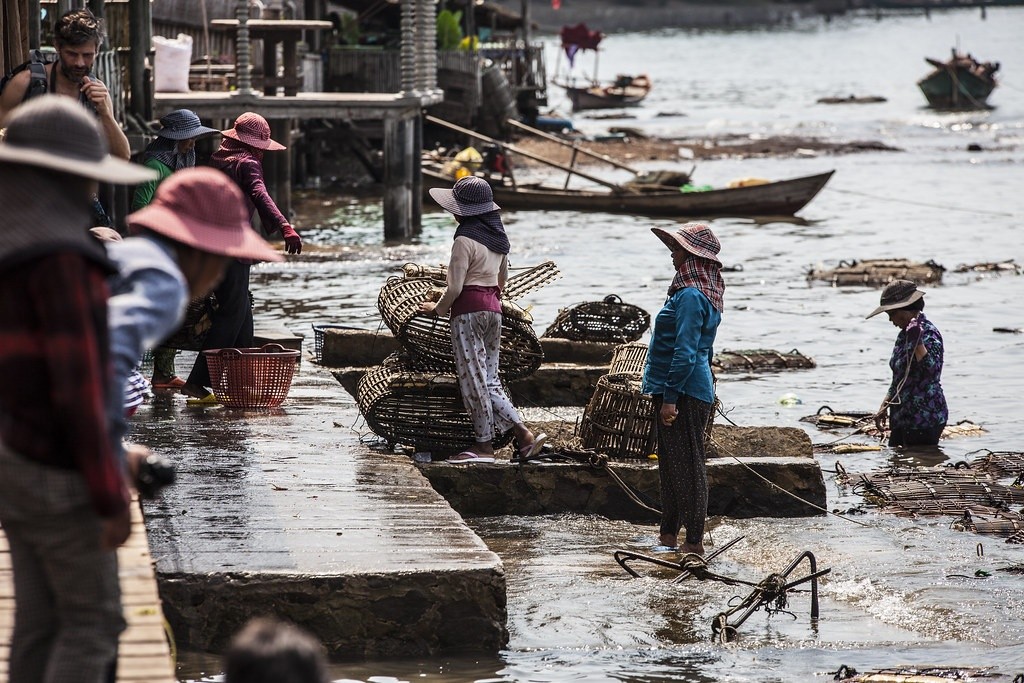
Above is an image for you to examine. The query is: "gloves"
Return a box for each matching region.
[281,225,303,257]
[907,318,925,346]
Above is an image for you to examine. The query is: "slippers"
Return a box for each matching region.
[521,432,547,458]
[187,392,217,403]
[445,451,495,464]
[153,376,187,389]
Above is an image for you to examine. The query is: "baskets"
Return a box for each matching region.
[202,344,301,408]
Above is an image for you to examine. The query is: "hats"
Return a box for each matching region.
[222,111,291,152]
[127,166,280,262]
[153,108,221,140]
[429,175,502,215]
[865,280,925,320]
[652,224,724,269]
[1,94,158,185]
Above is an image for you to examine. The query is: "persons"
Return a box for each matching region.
[180,112,302,404]
[90,168,287,499]
[419,176,547,464]
[866,280,948,446]
[641,224,725,554]
[131,109,220,389]
[0,95,161,683]
[0,8,131,161]
[224,616,329,683]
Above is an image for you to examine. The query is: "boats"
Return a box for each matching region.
[369,116,836,217]
[917,35,1000,111]
[550,23,651,112]
[817,94,887,105]
